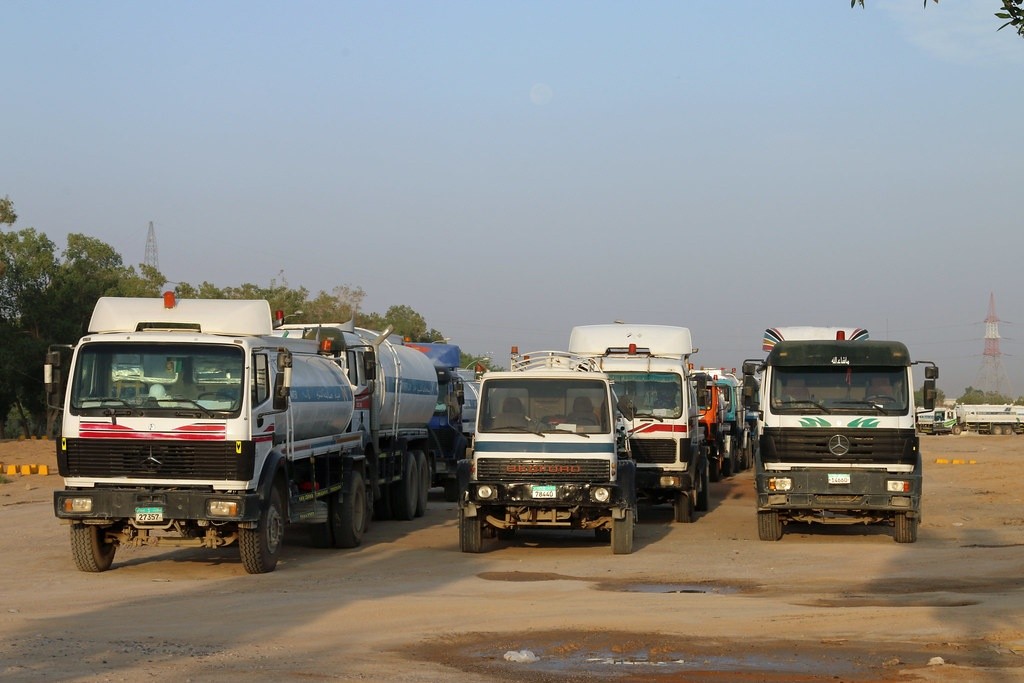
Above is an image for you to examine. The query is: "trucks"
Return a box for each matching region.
[412,321,940,543]
[446,345,638,554]
[44,289,372,576]
[274,306,439,519]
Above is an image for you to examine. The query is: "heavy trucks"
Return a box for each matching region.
[932,402,1024,435]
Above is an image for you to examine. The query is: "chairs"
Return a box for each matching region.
[493,397,527,427]
[782,376,811,402]
[112,365,148,398]
[865,373,894,398]
[654,389,675,410]
[569,396,600,425]
